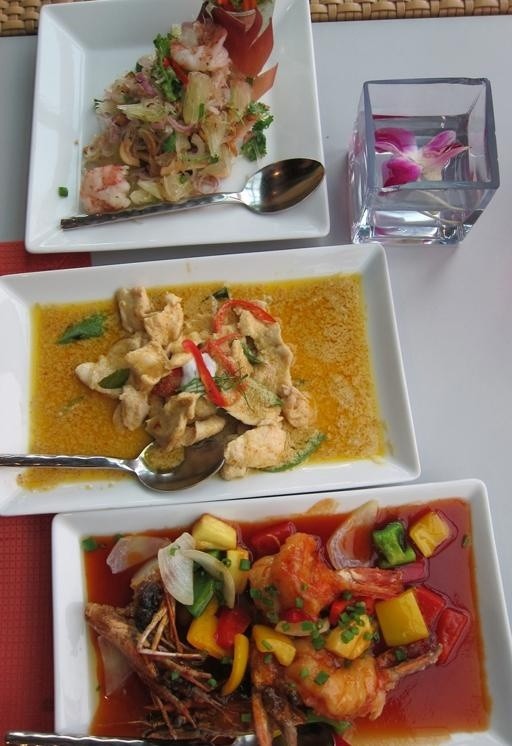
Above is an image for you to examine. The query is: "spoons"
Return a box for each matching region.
[61,158,325,231]
[5,721,336,746]
[0,436,225,492]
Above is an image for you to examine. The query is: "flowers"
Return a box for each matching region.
[372,120,466,191]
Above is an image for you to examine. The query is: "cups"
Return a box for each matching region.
[346,76,501,246]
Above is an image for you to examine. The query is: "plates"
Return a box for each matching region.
[0,243,421,517]
[50,478,512,746]
[24,0,331,254]
